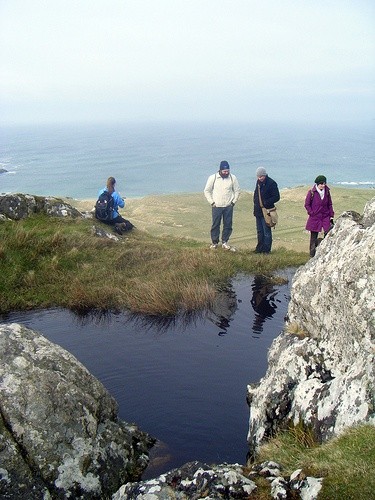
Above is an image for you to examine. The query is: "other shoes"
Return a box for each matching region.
[252,250,270,255]
[210,243,218,249]
[222,242,231,250]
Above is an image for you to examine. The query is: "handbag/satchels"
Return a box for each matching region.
[261,206,278,228]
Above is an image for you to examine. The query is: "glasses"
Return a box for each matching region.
[318,182,327,185]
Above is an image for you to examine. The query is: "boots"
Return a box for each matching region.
[114,222,126,235]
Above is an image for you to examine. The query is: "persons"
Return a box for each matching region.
[100,177,133,235]
[304,175,335,258]
[204,161,241,249]
[252,167,280,253]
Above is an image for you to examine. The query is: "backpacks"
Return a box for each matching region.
[94,190,115,218]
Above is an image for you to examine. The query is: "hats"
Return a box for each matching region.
[219,161,230,170]
[256,167,268,176]
[315,175,326,183]
